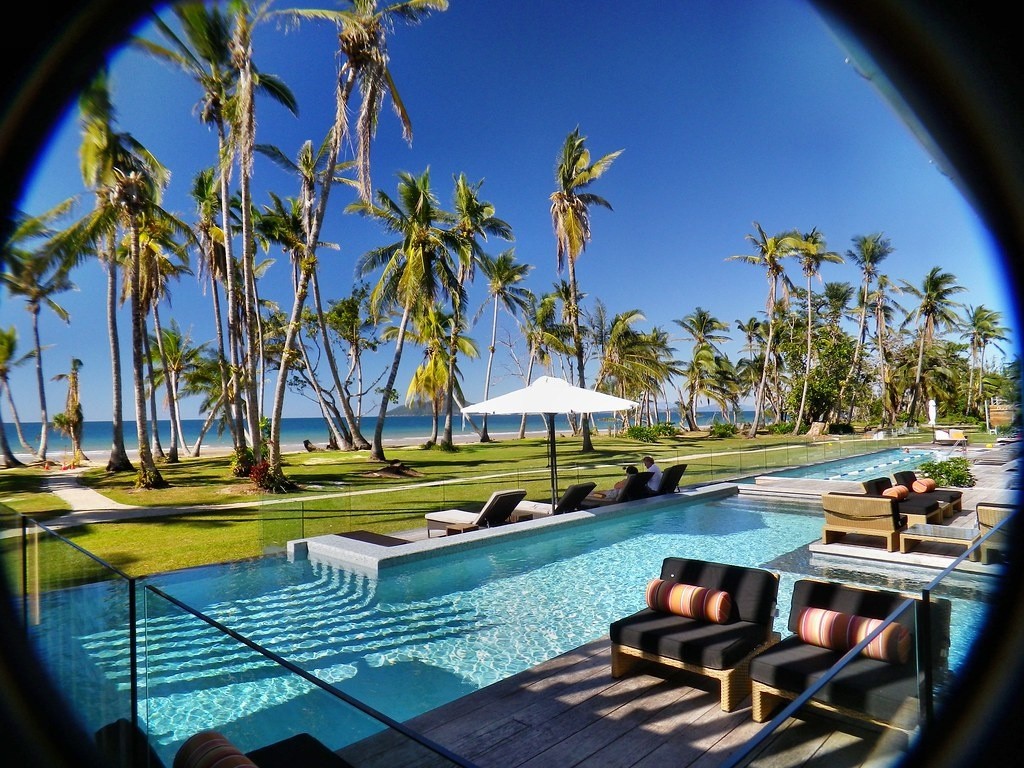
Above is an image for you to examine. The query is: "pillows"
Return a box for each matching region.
[883,485,909,501]
[794,605,911,666]
[173,731,261,768]
[645,579,731,625]
[912,477,936,494]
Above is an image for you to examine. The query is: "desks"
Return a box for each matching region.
[446,523,480,535]
[899,524,981,561]
[505,511,533,523]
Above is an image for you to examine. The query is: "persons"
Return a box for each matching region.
[902,448,909,453]
[588,466,638,498]
[644,457,662,492]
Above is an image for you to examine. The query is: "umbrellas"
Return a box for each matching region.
[928,400,936,438]
[461,376,639,516]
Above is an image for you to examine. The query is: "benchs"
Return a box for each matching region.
[934,430,967,446]
[750,580,952,749]
[821,492,908,552]
[610,557,779,711]
[976,502,1021,565]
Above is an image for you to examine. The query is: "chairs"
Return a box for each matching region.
[862,477,941,528]
[94,716,355,768]
[973,425,1024,489]
[895,471,963,518]
[514,482,597,516]
[579,471,656,508]
[425,488,528,538]
[645,464,689,497]
